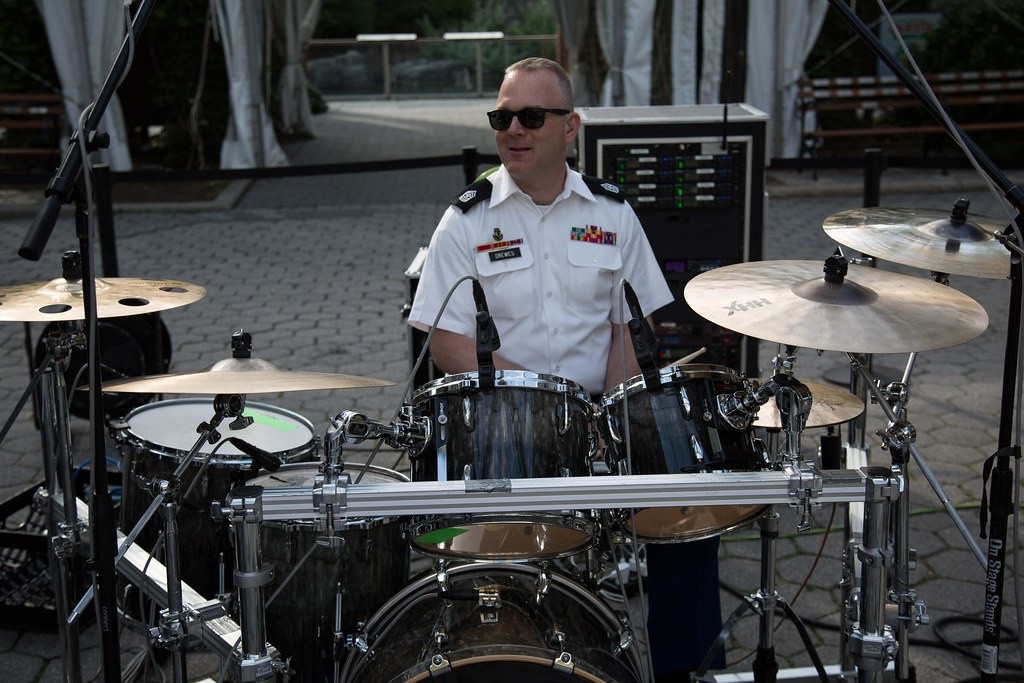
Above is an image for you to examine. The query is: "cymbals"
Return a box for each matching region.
[684,246,989,354]
[822,196,1010,280]
[0,252,209,322]
[74,329,399,395]
[743,374,866,428]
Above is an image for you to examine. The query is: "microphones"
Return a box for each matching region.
[624,281,656,347]
[471,279,501,352]
[230,437,281,472]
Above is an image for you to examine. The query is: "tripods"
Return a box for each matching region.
[691,509,828,683]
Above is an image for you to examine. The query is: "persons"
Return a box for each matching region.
[407,56,674,461]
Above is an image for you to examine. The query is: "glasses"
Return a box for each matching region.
[487,108,572,131]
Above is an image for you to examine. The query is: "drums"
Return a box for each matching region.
[339,563,647,682]
[597,364,775,544]
[223,462,414,683]
[113,397,323,637]
[403,368,594,562]
